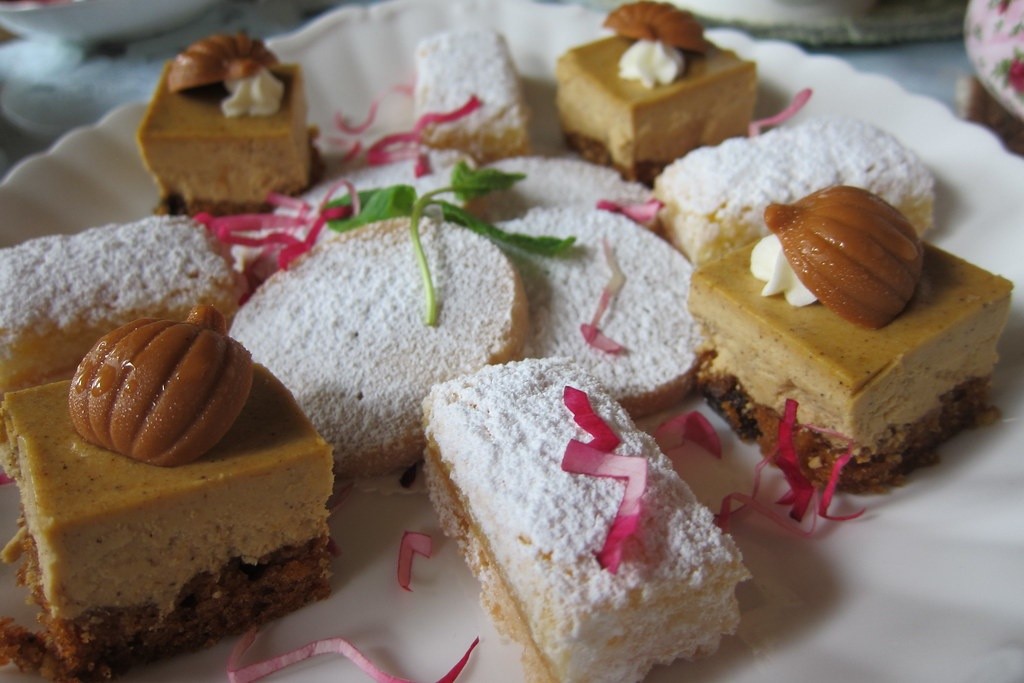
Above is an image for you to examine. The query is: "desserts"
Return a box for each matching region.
[0,0,1015,683]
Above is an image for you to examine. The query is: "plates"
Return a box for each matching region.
[0,1,307,144]
[0,0,1023,682]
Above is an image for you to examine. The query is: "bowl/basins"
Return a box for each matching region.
[0,1,203,44]
